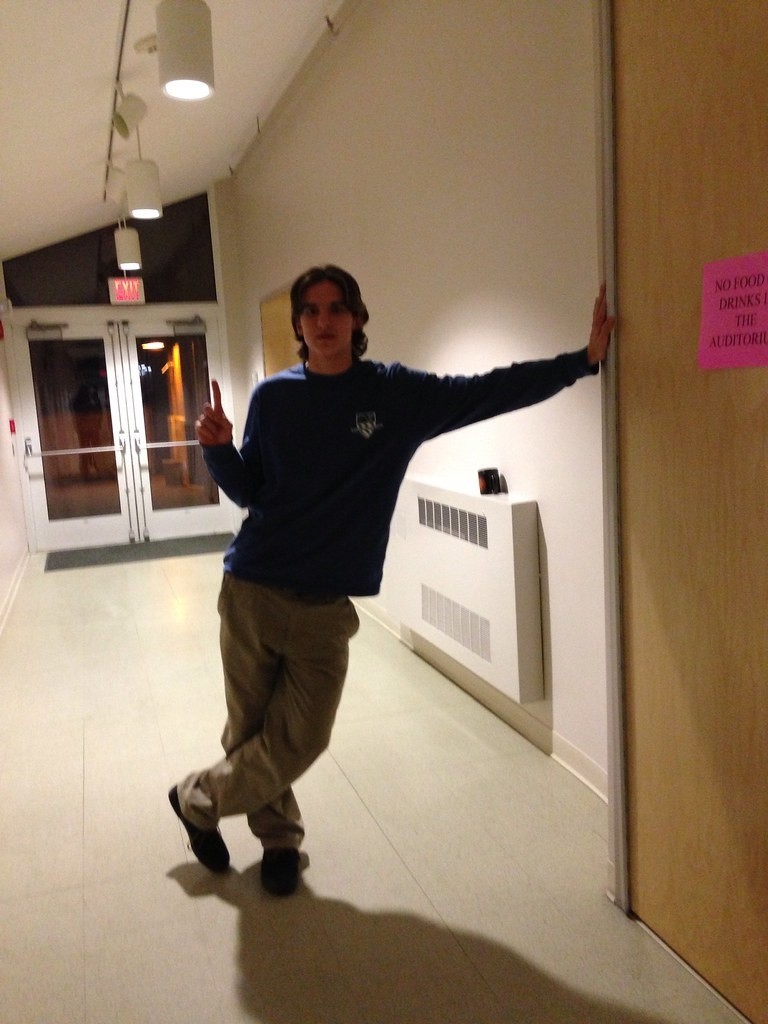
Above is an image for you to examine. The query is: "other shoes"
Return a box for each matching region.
[260,846,301,898]
[168,785,230,874]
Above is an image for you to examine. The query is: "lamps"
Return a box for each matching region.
[115,214,142,271]
[113,81,148,141]
[124,125,163,220]
[155,0,214,101]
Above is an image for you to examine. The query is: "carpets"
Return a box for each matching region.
[44,532,236,572]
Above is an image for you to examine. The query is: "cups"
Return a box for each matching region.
[478,468,500,495]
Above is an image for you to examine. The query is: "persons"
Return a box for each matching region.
[68,384,97,478]
[168,264,615,895]
